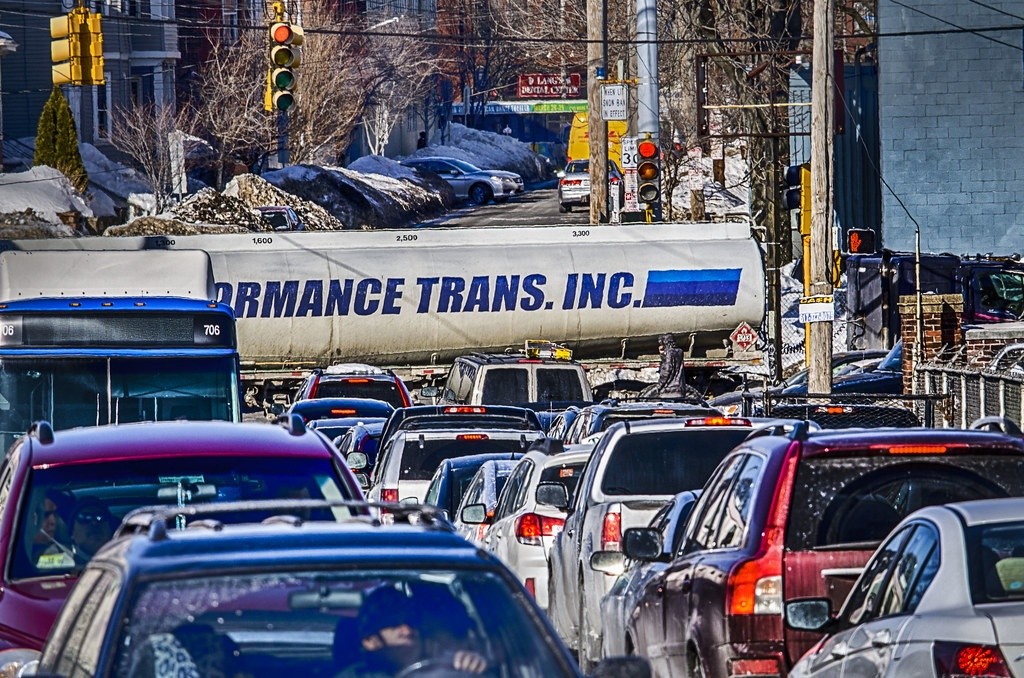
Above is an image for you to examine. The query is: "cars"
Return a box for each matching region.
[370,401,1024,678]
[398,156,525,205]
[17,497,655,678]
[707,348,887,407]
[782,340,904,395]
[337,423,390,497]
[557,159,626,213]
[421,349,595,407]
[0,420,373,678]
[285,397,396,432]
[305,419,389,446]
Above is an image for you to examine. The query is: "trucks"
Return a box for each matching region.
[1,250,242,487]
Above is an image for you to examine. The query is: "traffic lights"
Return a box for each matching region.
[637,137,661,204]
[847,228,876,256]
[785,161,812,210]
[48,16,105,87]
[264,21,303,114]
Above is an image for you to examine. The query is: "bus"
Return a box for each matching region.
[566,111,629,186]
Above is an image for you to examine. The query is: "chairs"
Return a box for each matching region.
[793,495,901,547]
[169,405,200,419]
[984,557,1024,602]
[127,631,254,678]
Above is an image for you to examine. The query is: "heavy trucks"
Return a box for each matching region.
[1,220,765,374]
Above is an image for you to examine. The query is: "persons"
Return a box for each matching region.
[656,333,685,401]
[438,114,447,129]
[417,131,427,149]
[25,491,113,574]
[334,585,489,678]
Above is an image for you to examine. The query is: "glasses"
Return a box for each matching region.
[74,511,109,525]
